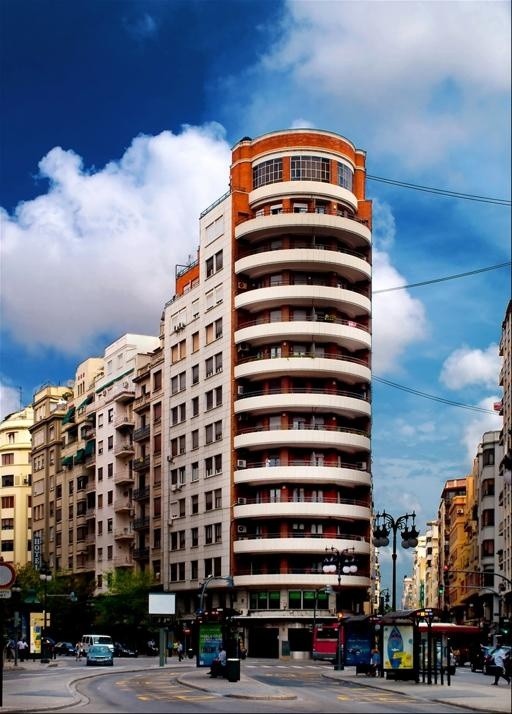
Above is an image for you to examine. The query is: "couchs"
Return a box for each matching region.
[439,566,449,594]
[228,576,234,588]
[325,584,331,596]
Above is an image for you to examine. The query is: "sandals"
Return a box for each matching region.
[312,622,480,666]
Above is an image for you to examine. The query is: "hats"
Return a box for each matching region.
[421,642,456,675]
[54,635,138,666]
[471,646,512,674]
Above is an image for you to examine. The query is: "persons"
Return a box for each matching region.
[7,636,16,650]
[5,635,16,662]
[488,641,510,685]
[76,640,84,660]
[206,646,227,678]
[18,637,29,662]
[173,638,178,657]
[176,639,184,662]
[148,638,152,656]
[167,637,173,656]
[150,637,155,646]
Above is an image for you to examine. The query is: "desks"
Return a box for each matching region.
[373,509,419,612]
[39,570,52,638]
[323,544,358,612]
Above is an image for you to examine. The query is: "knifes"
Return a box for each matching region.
[356,461,368,471]
[456,509,464,514]
[357,392,367,400]
[355,536,366,542]
[123,278,248,535]
